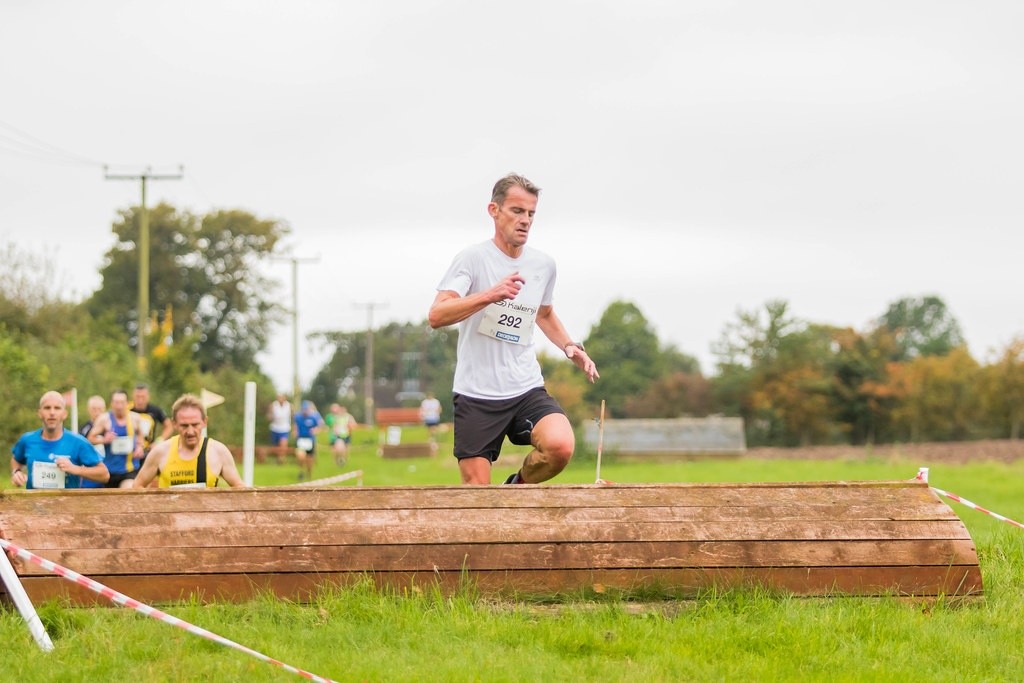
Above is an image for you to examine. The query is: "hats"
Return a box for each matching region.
[301,400,310,409]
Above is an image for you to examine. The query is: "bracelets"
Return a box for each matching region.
[13,468,21,474]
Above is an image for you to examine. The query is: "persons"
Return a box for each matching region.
[12,384,250,490]
[428,173,600,485]
[419,390,443,450]
[266,391,357,480]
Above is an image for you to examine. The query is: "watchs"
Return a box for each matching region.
[565,342,585,350]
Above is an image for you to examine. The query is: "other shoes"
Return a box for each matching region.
[501,473,518,484]
